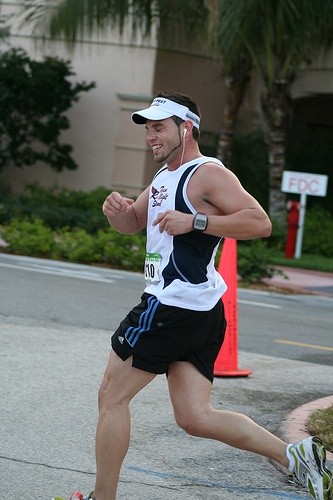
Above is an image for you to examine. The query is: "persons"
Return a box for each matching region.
[70,90,333,500]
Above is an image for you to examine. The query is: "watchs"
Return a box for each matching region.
[193,212,207,230]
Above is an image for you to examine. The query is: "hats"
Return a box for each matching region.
[130,95,200,129]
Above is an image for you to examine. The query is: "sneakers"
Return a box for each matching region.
[285,434,333,499]
[51,491,93,500]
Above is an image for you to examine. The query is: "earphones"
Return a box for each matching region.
[183,128,187,137]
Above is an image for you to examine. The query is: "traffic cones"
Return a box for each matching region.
[213,237,252,377]
